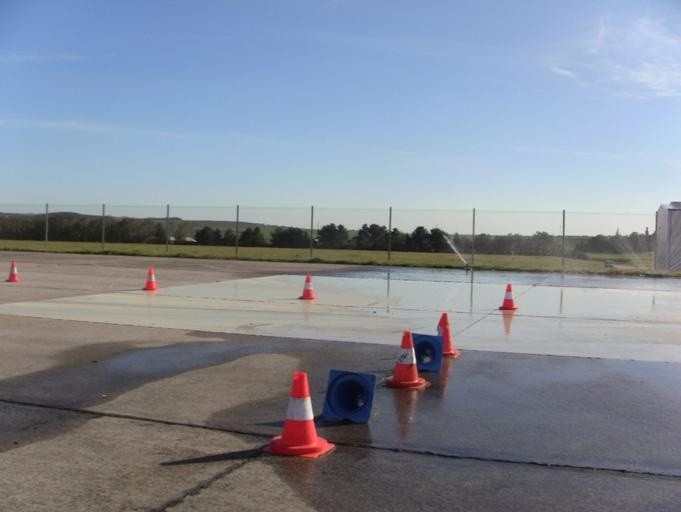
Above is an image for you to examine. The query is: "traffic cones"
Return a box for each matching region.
[497,284,519,311]
[5,262,21,282]
[391,386,417,439]
[262,371,336,459]
[380,332,432,391]
[296,276,319,299]
[501,310,514,336]
[438,356,452,397]
[433,313,460,359]
[142,267,159,291]
